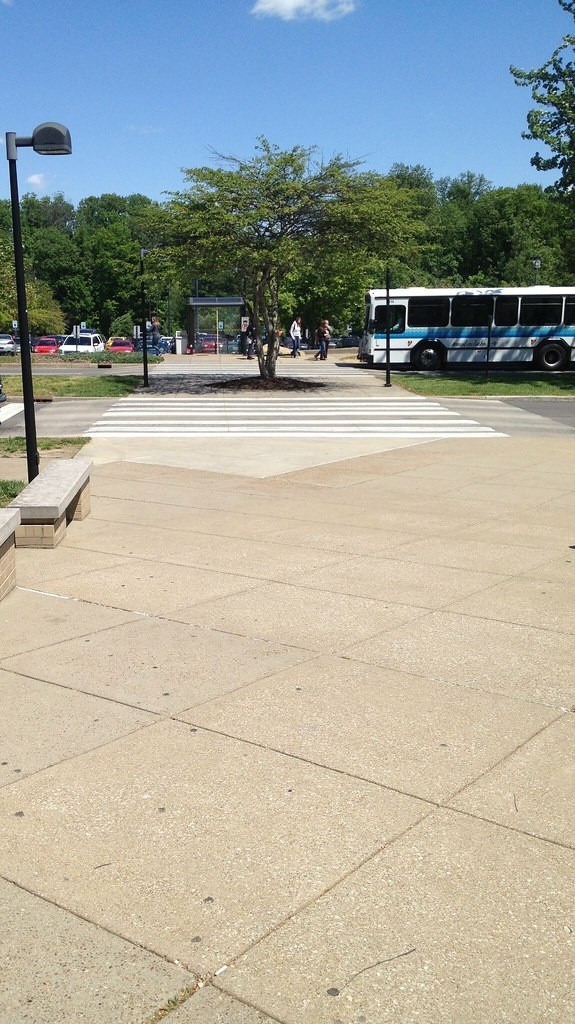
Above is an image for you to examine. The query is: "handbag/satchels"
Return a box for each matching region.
[246,336,253,344]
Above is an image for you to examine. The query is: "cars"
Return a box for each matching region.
[194,332,243,354]
[0,328,176,356]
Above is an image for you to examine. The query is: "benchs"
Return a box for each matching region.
[0,507,22,601]
[9,459,94,549]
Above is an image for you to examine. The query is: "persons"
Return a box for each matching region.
[390,312,404,331]
[246,325,256,359]
[148,316,164,356]
[289,316,301,358]
[314,320,332,359]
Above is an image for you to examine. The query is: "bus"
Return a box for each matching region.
[355,285,574,371]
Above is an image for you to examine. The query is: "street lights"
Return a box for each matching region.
[5,122,73,484]
[384,264,393,387]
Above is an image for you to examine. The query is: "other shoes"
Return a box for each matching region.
[247,357,254,359]
